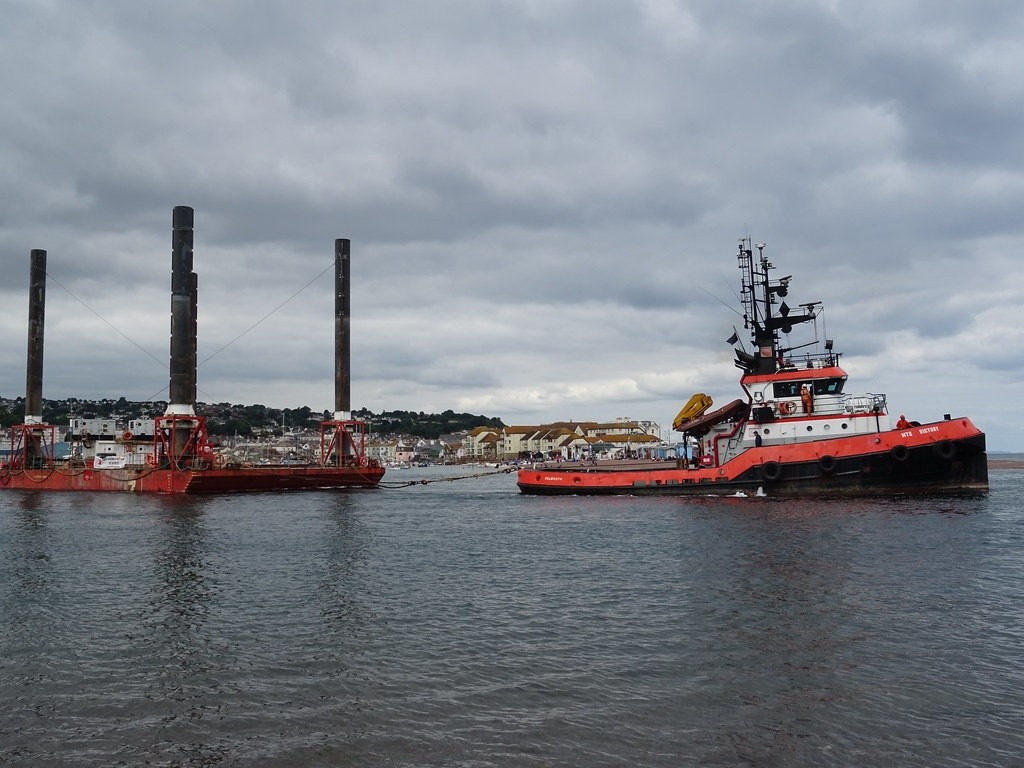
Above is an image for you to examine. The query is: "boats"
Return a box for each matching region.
[518,233,990,497]
[1,205,384,496]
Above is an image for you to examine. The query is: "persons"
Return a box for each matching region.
[800,383,813,418]
[897,415,915,430]
[754,431,762,447]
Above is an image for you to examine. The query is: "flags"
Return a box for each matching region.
[726,332,738,345]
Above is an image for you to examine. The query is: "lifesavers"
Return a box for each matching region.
[144,455,154,464]
[359,457,368,466]
[783,401,797,414]
[214,454,225,465]
[123,431,133,440]
